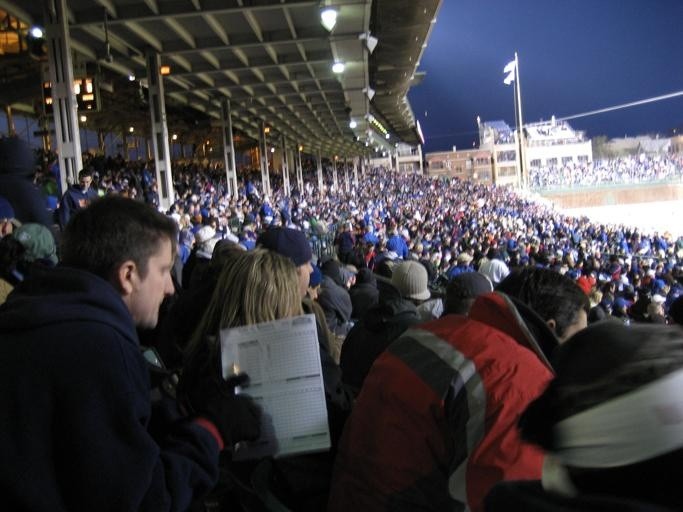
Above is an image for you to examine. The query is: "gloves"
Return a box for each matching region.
[201,376,262,445]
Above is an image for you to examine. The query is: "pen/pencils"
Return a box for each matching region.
[232,365,240,451]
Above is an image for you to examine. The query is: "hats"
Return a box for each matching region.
[448,272,492,298]
[196,226,216,243]
[310,259,339,287]
[357,269,377,285]
[457,253,473,262]
[3,224,59,271]
[256,228,311,265]
[393,261,431,299]
[614,297,628,306]
[647,294,666,315]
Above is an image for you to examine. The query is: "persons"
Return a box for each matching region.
[1,135,682,511]
